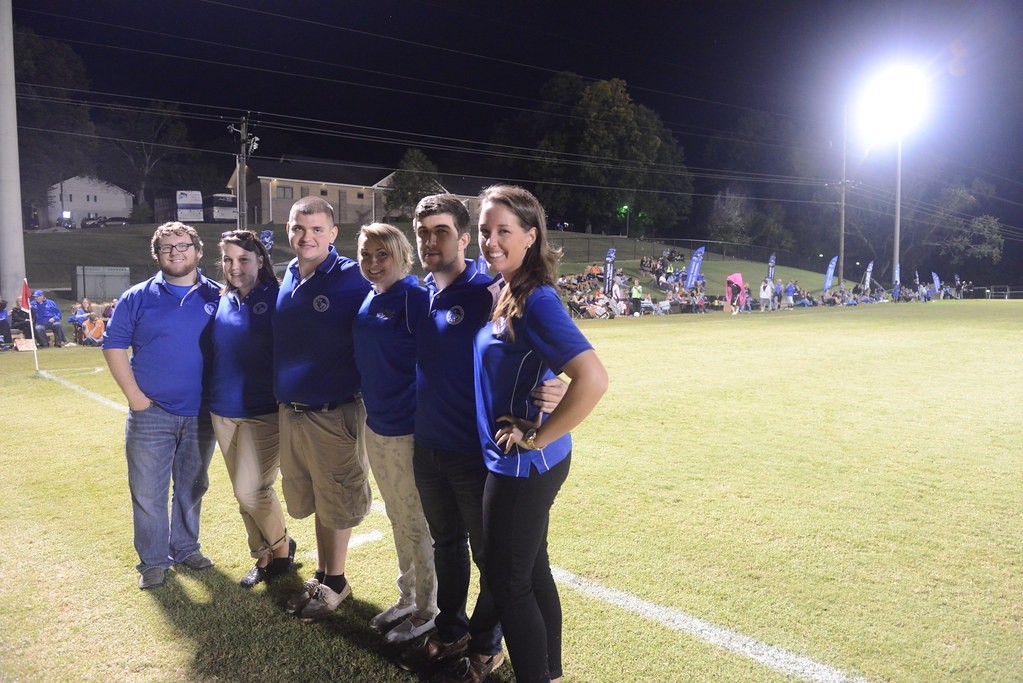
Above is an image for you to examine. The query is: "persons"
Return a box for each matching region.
[759,276,818,312]
[939,280,974,300]
[468,182,609,683]
[639,246,707,315]
[877,280,932,302]
[824,282,872,307]
[727,279,753,315]
[29,289,64,348]
[9,296,38,339]
[272,194,368,621]
[555,264,643,319]
[398,192,567,683]
[349,216,437,642]
[74,297,118,347]
[99,221,223,590]
[200,227,297,587]
[0,299,13,343]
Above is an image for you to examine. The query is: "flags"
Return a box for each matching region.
[20,278,31,313]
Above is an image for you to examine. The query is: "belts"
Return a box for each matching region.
[291,397,355,413]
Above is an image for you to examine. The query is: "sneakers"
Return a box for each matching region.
[298,576,351,621]
[438,648,504,683]
[399,632,471,672]
[286,578,320,612]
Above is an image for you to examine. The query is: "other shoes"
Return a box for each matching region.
[368,605,415,628]
[272,536,296,576]
[240,564,265,589]
[138,569,167,589]
[183,554,215,570]
[384,611,439,642]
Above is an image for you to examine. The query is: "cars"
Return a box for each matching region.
[56,217,75,228]
[80,217,128,228]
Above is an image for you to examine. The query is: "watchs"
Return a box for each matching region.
[525,428,548,450]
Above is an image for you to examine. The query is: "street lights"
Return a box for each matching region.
[623,199,630,238]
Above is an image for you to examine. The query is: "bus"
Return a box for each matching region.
[154,189,204,223]
[204,193,239,223]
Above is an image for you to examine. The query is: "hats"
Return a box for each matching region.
[32,290,44,297]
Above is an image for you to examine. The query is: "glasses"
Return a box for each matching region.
[221,230,255,241]
[159,243,194,253]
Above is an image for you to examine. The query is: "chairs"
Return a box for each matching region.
[642,298,658,316]
[10,328,25,350]
[745,299,760,310]
[821,296,832,306]
[71,301,111,347]
[659,300,672,315]
[567,301,587,319]
[704,295,726,311]
[45,329,55,347]
